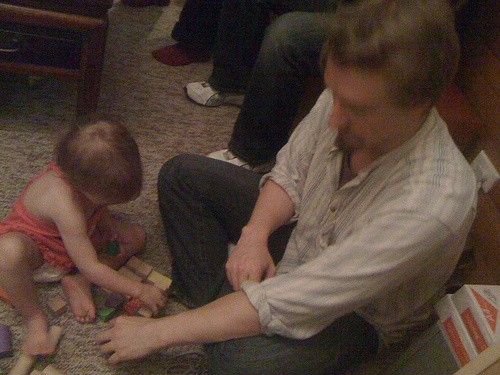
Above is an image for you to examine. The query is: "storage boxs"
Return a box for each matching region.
[434,284,500,367]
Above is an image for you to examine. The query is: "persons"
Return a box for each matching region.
[119,0,221,67]
[94,0,480,375]
[183,0,339,174]
[0,115,169,358]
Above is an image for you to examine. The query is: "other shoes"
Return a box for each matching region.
[150,44,213,66]
[185,81,247,109]
[205,149,277,174]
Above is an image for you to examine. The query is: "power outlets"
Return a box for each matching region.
[470,150,500,194]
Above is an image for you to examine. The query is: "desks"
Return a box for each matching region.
[1,0,108,115]
